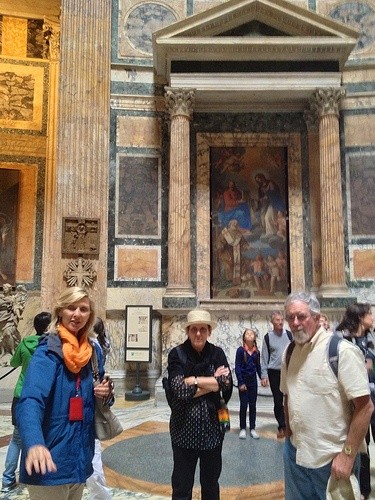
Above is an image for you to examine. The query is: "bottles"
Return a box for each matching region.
[218,389,230,431]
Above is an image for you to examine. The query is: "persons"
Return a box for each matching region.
[278,291,375,500]
[0,312,111,493]
[162,310,233,500]
[14,287,115,500]
[0,283,23,357]
[235,329,267,439]
[261,303,375,500]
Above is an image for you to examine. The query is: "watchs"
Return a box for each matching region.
[341,447,354,457]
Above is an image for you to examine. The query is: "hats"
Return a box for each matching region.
[181,309,218,331]
[326,472,361,500]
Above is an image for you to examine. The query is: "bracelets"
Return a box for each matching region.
[194,375,198,386]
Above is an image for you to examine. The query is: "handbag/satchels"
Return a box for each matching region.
[89,339,123,441]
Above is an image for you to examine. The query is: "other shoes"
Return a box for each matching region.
[1,479,17,492]
[239,429,246,439]
[277,428,284,438]
[250,429,260,439]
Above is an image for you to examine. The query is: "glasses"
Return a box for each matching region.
[285,312,312,321]
